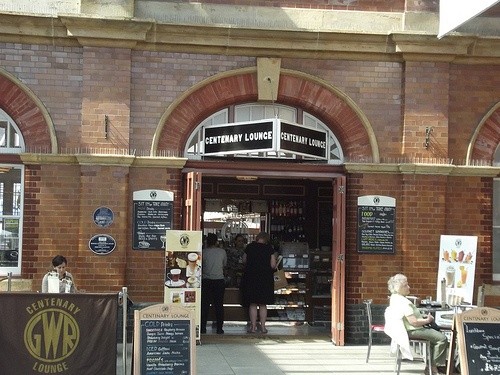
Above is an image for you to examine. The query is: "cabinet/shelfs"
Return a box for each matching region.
[259,256,332,326]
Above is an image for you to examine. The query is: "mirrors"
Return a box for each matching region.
[202,198,268,292]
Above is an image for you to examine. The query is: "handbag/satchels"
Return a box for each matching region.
[272,266,287,291]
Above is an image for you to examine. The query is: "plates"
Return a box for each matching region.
[165,279,185,287]
[186,265,200,277]
[186,282,200,288]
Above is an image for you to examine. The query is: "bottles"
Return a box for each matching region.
[271,201,304,244]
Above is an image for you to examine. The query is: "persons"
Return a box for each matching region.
[240,231,277,334]
[229,235,245,257]
[44,255,75,292]
[201,232,229,334]
[387,274,447,375]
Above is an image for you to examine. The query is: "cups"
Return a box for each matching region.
[166,269,181,281]
[188,276,197,284]
[187,253,198,272]
[446,266,467,288]
[442,249,472,262]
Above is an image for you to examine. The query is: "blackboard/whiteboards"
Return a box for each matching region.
[447,307,500,375]
[131,304,196,375]
[358,206,395,253]
[133,201,172,249]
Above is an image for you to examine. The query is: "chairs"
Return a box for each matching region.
[364,298,385,364]
[385,293,434,375]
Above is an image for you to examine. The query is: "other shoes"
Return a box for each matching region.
[425,367,444,375]
[201,326,206,331]
[217,328,224,333]
